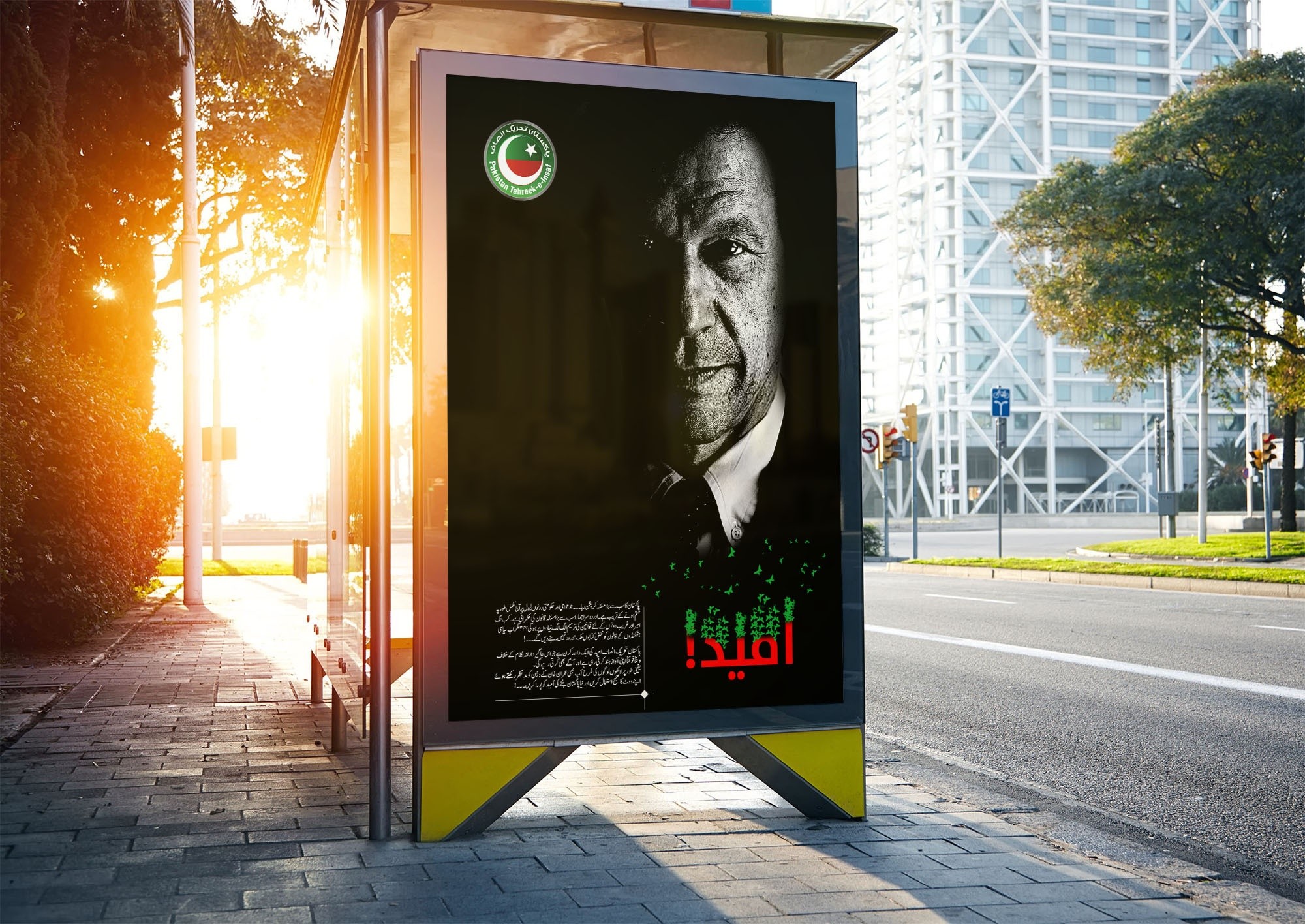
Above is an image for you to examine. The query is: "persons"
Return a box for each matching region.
[566,104,843,709]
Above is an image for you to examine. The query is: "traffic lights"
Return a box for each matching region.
[879,424,898,464]
[1249,449,1263,471]
[899,403,917,443]
[1261,433,1276,465]
